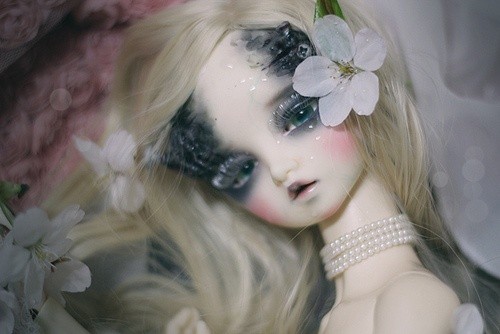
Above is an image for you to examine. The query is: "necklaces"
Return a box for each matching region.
[319,214,417,280]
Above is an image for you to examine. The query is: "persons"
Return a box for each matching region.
[40,0,500,334]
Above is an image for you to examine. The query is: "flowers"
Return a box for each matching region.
[75,131,145,215]
[292,0,386,127]
[0,181,92,334]
